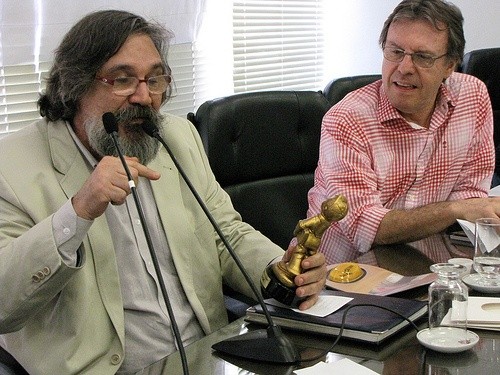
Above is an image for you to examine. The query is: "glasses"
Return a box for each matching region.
[94,74,172,96]
[382,46,448,69]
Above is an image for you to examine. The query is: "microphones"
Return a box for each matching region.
[102,112,189,375]
[141,119,302,363]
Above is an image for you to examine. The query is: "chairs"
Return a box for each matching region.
[187,92,334,304]
[319,74,383,105]
[461,48,500,190]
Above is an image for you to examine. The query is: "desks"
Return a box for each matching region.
[132,223,500,375]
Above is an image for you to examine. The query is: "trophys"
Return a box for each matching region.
[260,193,348,309]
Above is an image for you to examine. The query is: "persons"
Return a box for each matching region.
[0,11,328,375]
[304,0,500,267]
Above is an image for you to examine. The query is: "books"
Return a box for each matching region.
[244,290,428,350]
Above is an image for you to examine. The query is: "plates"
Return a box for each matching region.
[462,274,500,293]
[416,327,479,353]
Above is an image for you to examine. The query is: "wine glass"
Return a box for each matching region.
[473,218,500,286]
[428,262,468,343]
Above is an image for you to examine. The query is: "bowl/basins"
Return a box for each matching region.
[448,258,473,273]
